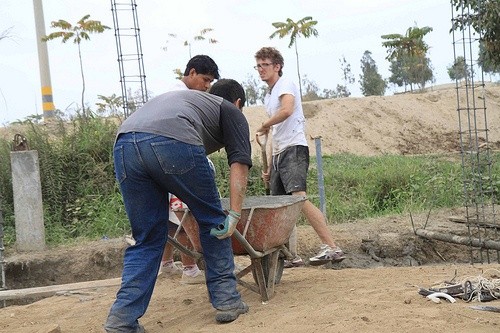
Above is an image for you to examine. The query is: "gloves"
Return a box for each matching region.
[210,209,241,240]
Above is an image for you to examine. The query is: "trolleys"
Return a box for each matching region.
[168,196,310,300]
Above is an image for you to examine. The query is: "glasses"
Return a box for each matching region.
[254,63,273,70]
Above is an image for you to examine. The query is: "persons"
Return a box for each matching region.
[155,54,221,285]
[102,77,253,333]
[254,46,347,268]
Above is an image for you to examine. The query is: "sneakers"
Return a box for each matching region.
[158,264,183,280]
[105,325,145,333]
[284,255,304,268]
[215,302,248,321]
[309,241,346,266]
[182,272,206,284]
